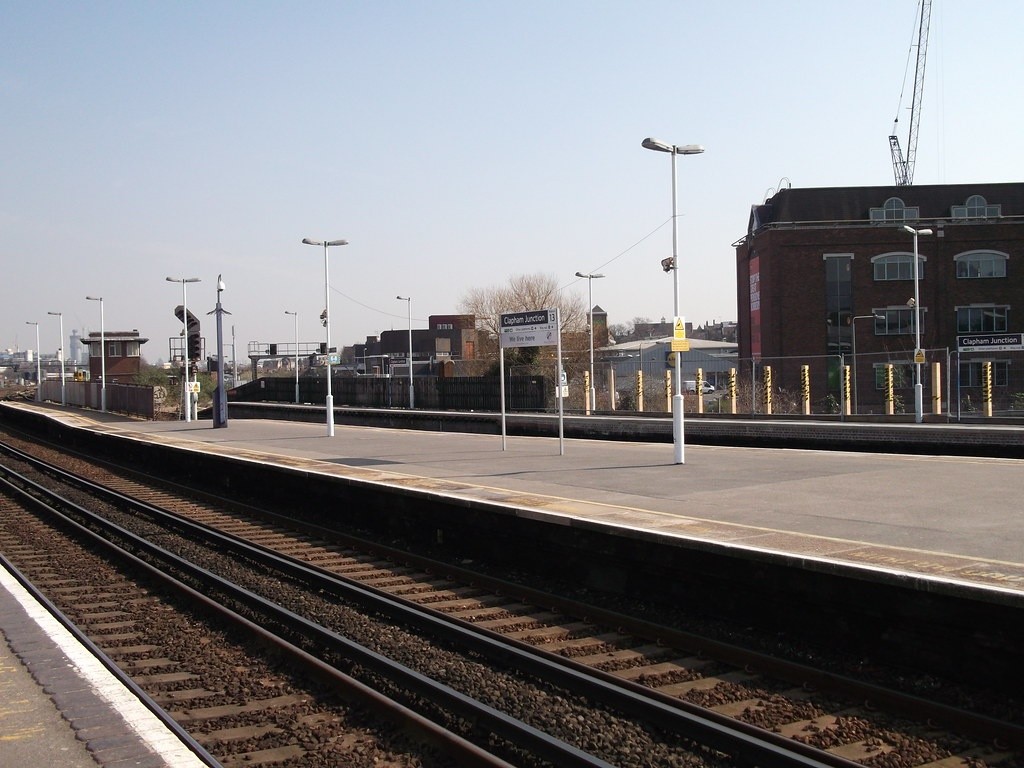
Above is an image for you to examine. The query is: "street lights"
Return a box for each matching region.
[26,320,43,403]
[302,237,351,438]
[285,311,300,404]
[574,270,608,414]
[48,311,66,408]
[902,222,932,423]
[205,273,233,429]
[85,295,106,412]
[165,275,203,425]
[643,137,707,465]
[396,295,416,409]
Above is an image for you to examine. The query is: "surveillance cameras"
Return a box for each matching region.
[217,281,225,291]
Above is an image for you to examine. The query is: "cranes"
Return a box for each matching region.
[886,0,934,186]
